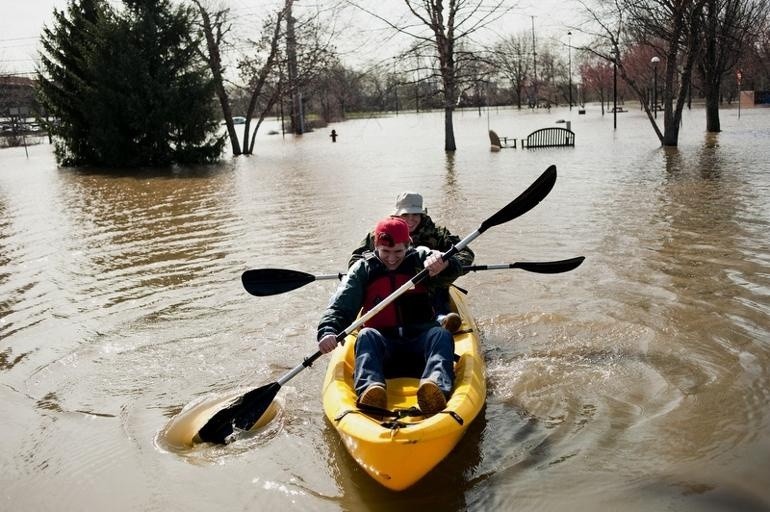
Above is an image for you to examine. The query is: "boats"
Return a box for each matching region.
[319,283,490,497]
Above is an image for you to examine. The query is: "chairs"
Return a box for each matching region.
[488,128,516,149]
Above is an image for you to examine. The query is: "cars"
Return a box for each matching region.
[0,119,61,137]
[607,106,629,113]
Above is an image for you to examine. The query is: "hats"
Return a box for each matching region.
[391,193,428,215]
[374,217,410,247]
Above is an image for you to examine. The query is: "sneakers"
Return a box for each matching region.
[443,312,462,334]
[359,386,386,415]
[416,382,447,419]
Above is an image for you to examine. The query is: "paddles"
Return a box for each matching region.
[184,164,557,448]
[242,256,587,297]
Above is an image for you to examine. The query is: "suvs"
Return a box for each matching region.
[220,116,246,125]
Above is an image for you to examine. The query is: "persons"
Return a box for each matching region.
[348,192,478,336]
[316,217,457,422]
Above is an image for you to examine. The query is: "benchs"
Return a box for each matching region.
[522,127,579,148]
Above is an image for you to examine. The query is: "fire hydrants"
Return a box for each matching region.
[328,128,339,142]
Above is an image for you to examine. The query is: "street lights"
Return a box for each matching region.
[609,44,619,129]
[567,31,573,111]
[650,56,661,120]
